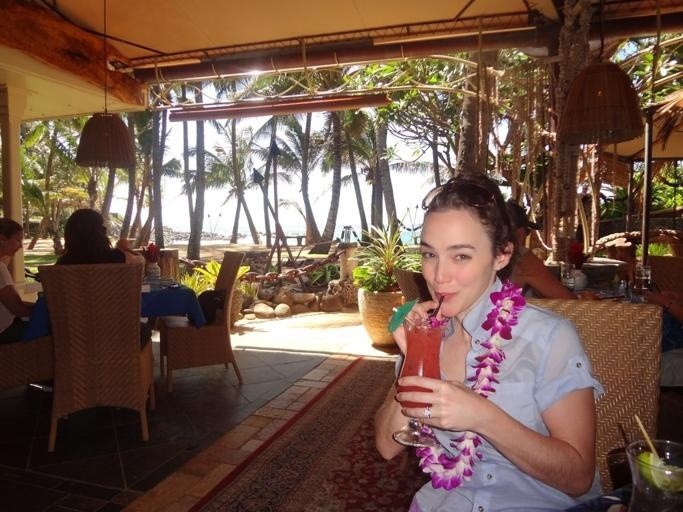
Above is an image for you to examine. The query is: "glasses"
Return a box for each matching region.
[422,183,497,211]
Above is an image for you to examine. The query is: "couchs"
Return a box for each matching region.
[593,257,633,294]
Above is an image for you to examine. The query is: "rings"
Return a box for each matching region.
[424,406,430,419]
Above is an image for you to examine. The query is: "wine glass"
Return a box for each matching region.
[394,319,448,447]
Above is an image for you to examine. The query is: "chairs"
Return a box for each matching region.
[580,263,619,289]
[526,298,664,495]
[127,248,181,284]
[545,266,561,281]
[646,255,683,292]
[644,290,683,323]
[38,256,156,452]
[159,251,246,392]
[0,334,54,391]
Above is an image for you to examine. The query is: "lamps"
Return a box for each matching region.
[75,0,136,168]
[556,0,645,145]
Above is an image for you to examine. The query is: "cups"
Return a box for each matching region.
[625,439,683,512]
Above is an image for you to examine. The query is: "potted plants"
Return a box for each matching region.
[346,203,406,348]
[393,253,433,301]
[192,259,251,327]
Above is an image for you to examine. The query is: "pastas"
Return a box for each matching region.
[639,452,682,494]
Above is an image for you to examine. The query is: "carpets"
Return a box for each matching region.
[187,356,432,511]
[1,339,331,511]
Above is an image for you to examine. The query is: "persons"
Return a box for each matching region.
[497,203,579,299]
[374,170,605,512]
[0,217,35,344]
[55,209,145,263]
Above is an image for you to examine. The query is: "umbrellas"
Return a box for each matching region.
[594,88,683,161]
